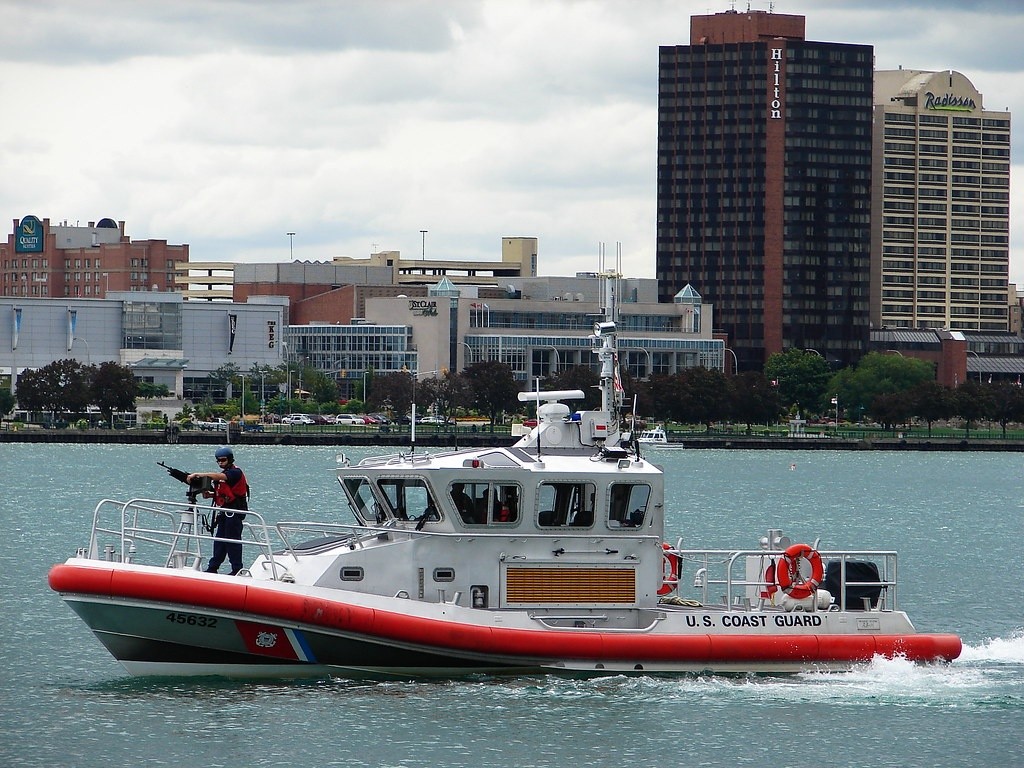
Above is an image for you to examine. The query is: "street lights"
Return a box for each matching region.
[69,333,984,414]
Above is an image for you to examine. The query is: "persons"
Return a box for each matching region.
[185,447,248,577]
[424,483,474,526]
[479,487,511,525]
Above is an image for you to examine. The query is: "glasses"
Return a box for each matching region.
[216,458,226,462]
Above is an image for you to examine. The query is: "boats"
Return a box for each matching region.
[47,237,963,685]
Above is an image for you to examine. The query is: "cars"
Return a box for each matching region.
[3,412,1021,434]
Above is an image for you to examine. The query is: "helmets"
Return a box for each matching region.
[214,448,234,457]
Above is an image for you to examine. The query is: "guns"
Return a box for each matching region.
[156,461,216,491]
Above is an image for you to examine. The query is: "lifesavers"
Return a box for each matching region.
[656,543,679,595]
[757,556,799,601]
[776,544,824,599]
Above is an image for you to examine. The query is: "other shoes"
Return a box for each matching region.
[204,566,218,573]
[227,570,239,576]
